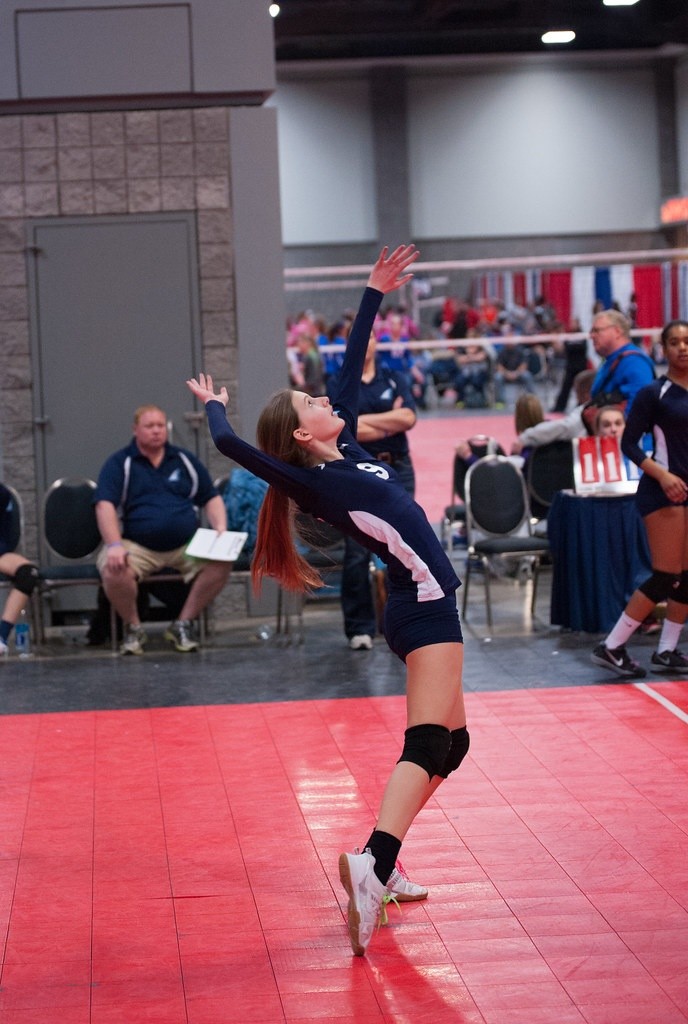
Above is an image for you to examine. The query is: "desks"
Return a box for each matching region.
[549,483,654,635]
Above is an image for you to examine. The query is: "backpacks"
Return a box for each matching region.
[217,467,270,556]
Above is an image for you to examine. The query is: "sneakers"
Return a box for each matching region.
[590,640,647,678]
[650,648,688,675]
[120,620,150,656]
[164,617,201,653]
[354,846,428,902]
[338,846,403,957]
[349,634,374,650]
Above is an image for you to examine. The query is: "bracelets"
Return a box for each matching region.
[108,542,120,547]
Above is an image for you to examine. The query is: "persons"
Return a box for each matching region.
[591,320,688,677]
[286,297,589,412]
[95,405,233,656]
[186,243,470,954]
[597,406,627,435]
[458,309,659,530]
[341,323,417,649]
[593,294,637,328]
[0,483,40,656]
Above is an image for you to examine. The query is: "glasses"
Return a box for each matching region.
[591,324,618,332]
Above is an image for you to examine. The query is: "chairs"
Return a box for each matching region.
[461,454,551,628]
[519,435,577,513]
[121,500,203,644]
[0,482,38,645]
[289,502,379,644]
[438,434,533,568]
[203,473,282,633]
[34,471,118,653]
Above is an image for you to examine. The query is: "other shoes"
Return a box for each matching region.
[0,636,10,660]
